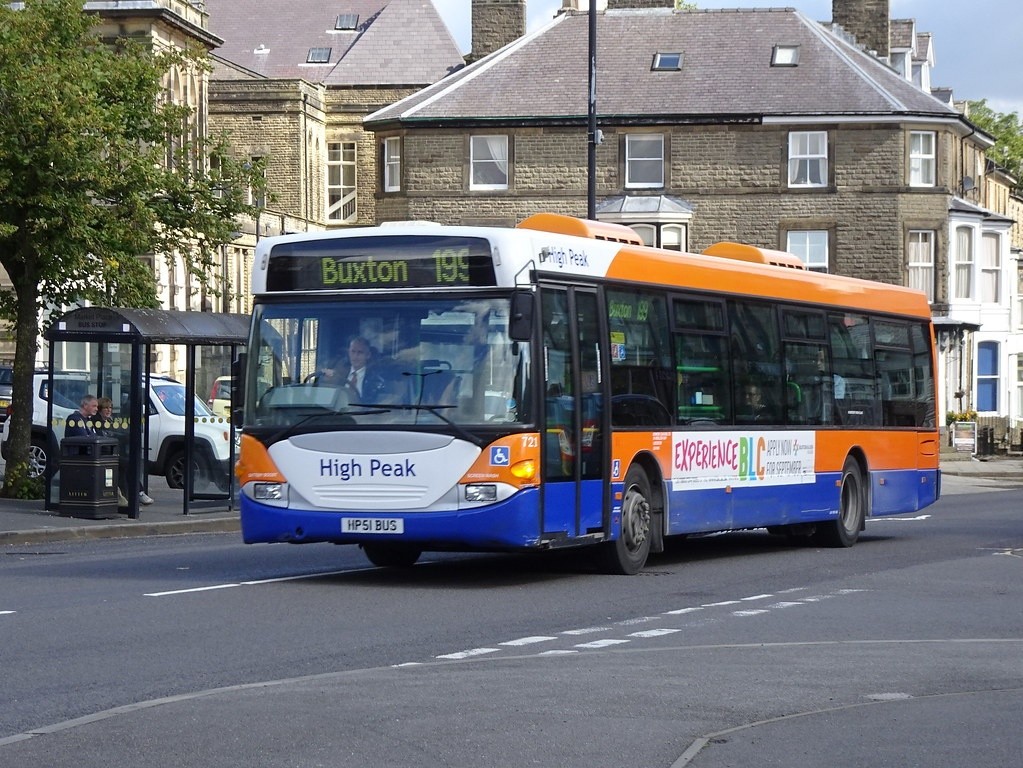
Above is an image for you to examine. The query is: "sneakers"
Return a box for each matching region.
[133,491,155,505]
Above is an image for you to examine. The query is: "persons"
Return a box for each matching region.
[321,335,389,402]
[64,395,144,513]
[734,381,777,424]
[91,396,155,505]
[808,349,846,424]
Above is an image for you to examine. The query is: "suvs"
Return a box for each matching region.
[0,367,67,429]
[0,371,242,498]
[207,376,274,426]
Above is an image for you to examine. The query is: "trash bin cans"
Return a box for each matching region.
[58,436,118,520]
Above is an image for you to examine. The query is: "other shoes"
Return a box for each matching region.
[115,496,140,513]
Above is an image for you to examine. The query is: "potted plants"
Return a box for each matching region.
[946,409,978,438]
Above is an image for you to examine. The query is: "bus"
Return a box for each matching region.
[232,213,941,576]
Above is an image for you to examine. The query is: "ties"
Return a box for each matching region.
[349,372,357,389]
